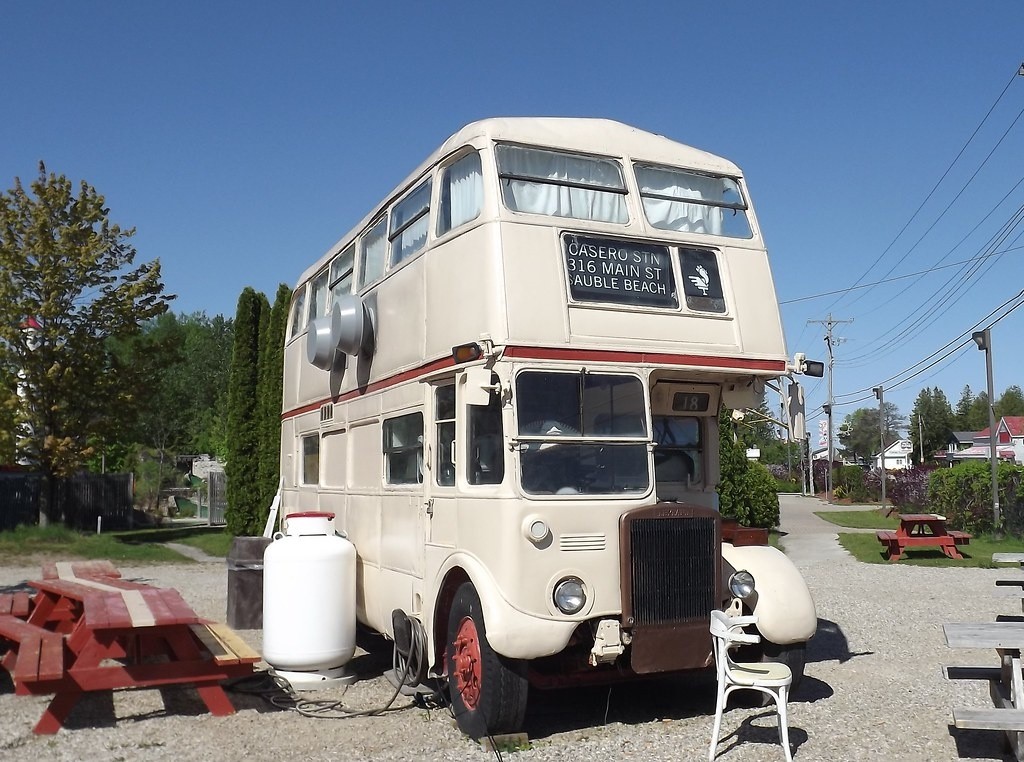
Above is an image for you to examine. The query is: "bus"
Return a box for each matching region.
[278,117,825,745]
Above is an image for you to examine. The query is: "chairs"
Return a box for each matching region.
[708,609,793,762]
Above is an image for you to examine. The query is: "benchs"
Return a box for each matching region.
[188,619,263,716]
[953,707,1024,762]
[946,530,973,560]
[875,530,900,561]
[0,591,66,736]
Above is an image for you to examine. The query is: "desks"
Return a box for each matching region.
[991,552,1024,571]
[30,559,121,611]
[889,513,964,561]
[942,622,1024,708]
[26,575,154,695]
[33,588,236,737]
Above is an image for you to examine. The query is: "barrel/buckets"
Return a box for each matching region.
[263,512,357,671]
